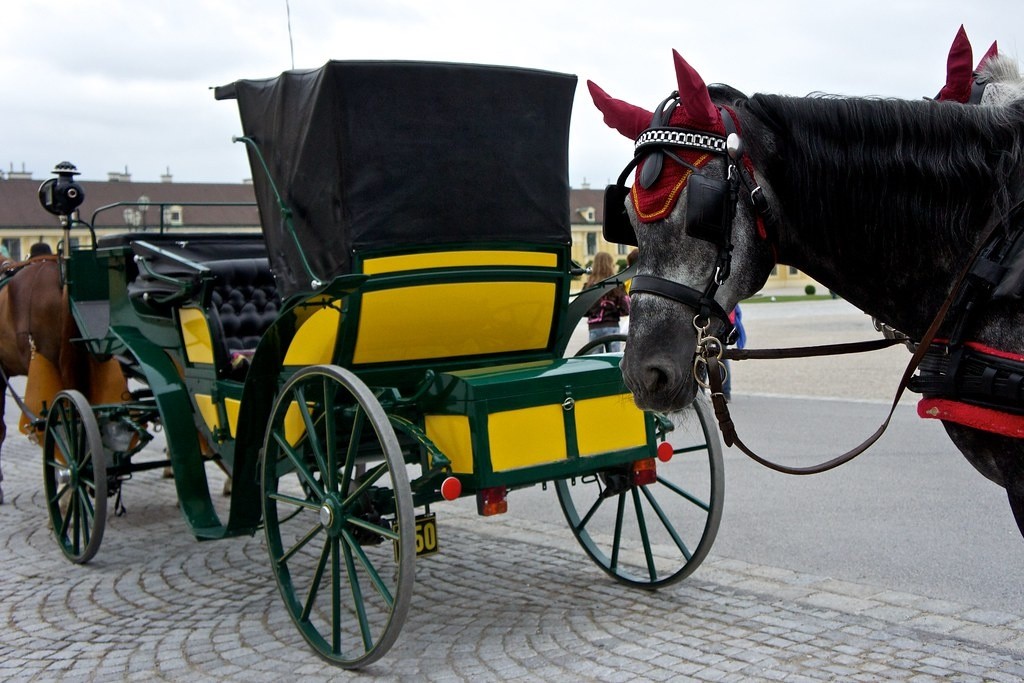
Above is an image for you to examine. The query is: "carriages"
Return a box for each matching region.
[0,58,726,670]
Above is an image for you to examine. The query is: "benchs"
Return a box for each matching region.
[193,258,281,361]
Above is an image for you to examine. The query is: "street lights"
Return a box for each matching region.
[123,195,151,232]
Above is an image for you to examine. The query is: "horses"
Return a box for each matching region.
[586,25,1024,538]
[0,249,112,500]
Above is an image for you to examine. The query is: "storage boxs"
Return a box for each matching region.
[422,354,658,487]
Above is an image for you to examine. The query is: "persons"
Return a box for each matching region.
[582,252,630,354]
[719,305,746,401]
